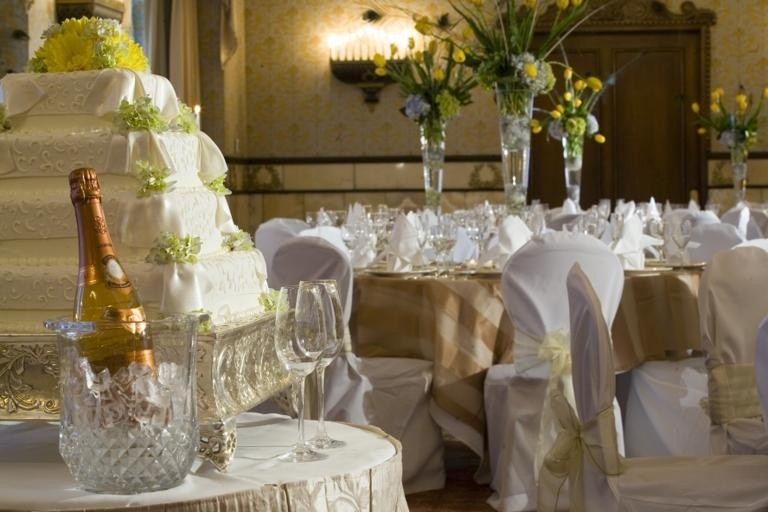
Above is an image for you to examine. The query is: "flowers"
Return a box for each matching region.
[691,86,768,164]
[414,0,589,118]
[373,38,479,124]
[528,37,606,146]
[28,16,149,79]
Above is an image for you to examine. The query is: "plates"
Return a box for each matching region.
[645,262,705,267]
[364,268,436,275]
[624,268,673,276]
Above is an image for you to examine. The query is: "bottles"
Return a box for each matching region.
[68,167,157,425]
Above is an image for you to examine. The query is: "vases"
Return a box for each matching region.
[731,144,748,202]
[419,125,444,211]
[495,79,534,204]
[560,136,583,202]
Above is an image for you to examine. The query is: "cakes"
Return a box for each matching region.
[0,16,289,337]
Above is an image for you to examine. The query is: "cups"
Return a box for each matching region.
[43,314,205,494]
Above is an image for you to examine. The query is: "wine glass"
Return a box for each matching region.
[300,203,664,280]
[671,218,691,274]
[295,280,344,448]
[274,285,326,461]
